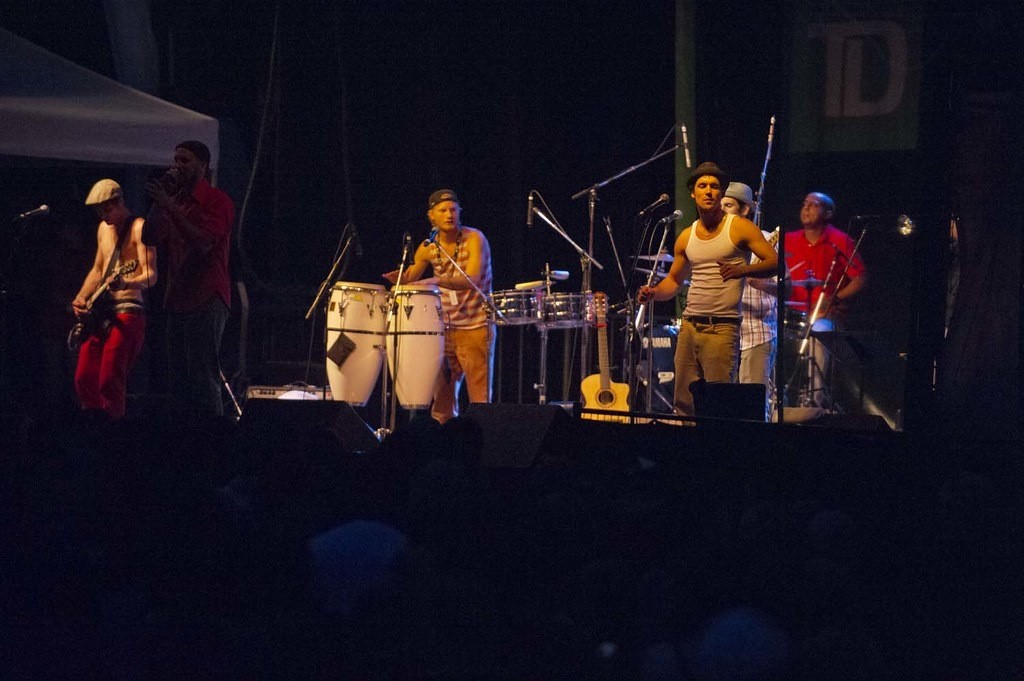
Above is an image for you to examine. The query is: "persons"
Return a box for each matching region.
[781,192,867,410]
[72,179,158,417]
[638,162,779,418]
[720,182,792,385]
[140,140,236,417]
[382,188,497,424]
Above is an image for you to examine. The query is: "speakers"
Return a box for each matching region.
[461,400,573,473]
[239,385,379,458]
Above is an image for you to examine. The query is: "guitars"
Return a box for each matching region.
[751,225,780,264]
[67,256,140,361]
[578,291,631,424]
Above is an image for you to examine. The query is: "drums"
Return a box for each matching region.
[536,291,608,331]
[384,283,446,410]
[783,307,808,333]
[323,280,390,407]
[489,288,547,326]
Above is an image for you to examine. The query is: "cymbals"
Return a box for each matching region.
[784,300,812,305]
[628,254,674,262]
[634,267,691,286]
[791,277,837,288]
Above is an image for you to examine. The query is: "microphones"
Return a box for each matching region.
[158,168,182,183]
[767,115,775,143]
[659,210,683,224]
[350,223,361,254]
[639,193,670,215]
[21,205,51,218]
[681,124,691,168]
[854,213,883,220]
[527,193,534,226]
[423,227,440,247]
[405,232,415,264]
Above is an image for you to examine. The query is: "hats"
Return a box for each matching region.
[725,182,755,213]
[687,162,730,191]
[86,178,121,204]
[428,189,460,209]
[175,140,211,167]
